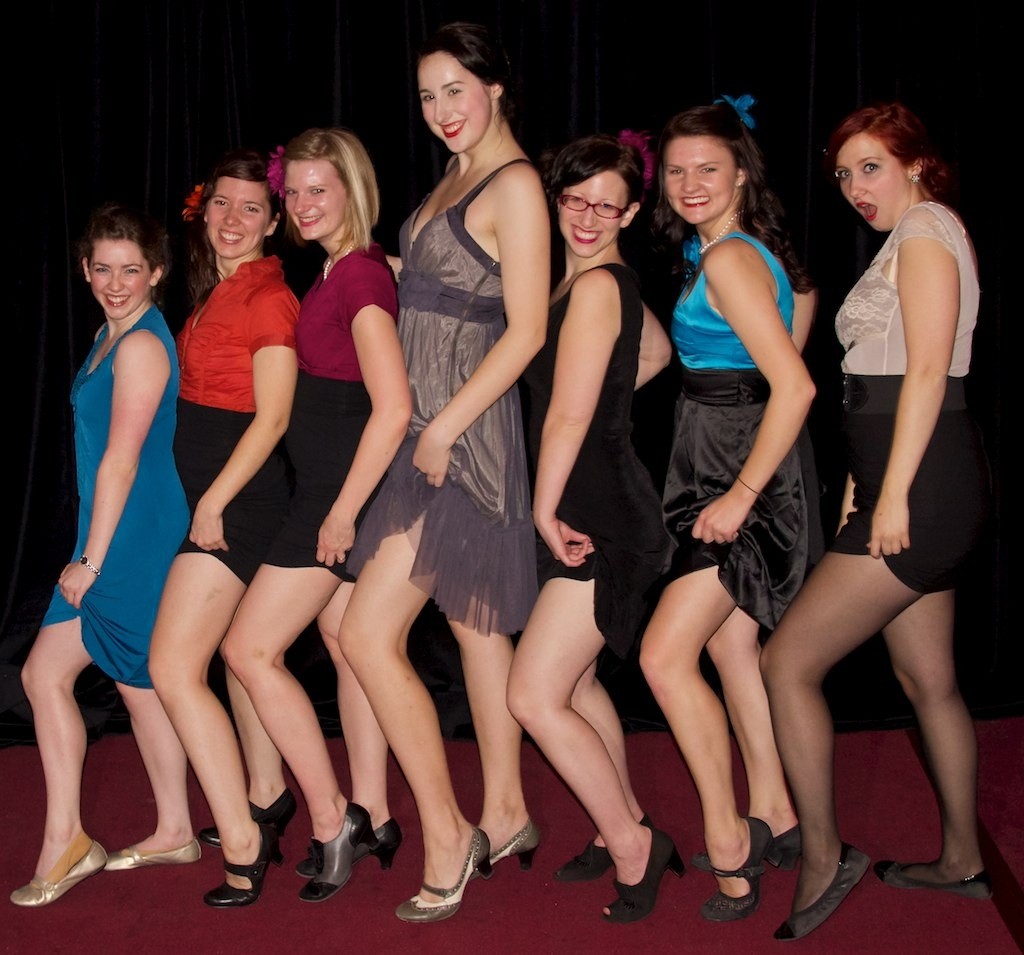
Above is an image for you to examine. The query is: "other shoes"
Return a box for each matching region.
[772,841,872,942]
[873,859,993,900]
[10,839,108,907]
[105,837,203,871]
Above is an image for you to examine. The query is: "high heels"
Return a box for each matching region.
[601,827,686,922]
[198,787,297,848]
[394,826,495,923]
[295,816,403,879]
[699,816,783,921]
[690,823,801,871]
[299,800,380,903]
[203,822,285,908]
[554,812,656,880]
[467,814,539,881]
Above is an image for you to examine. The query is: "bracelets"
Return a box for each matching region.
[737,476,760,494]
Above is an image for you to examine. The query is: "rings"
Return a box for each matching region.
[337,555,345,561]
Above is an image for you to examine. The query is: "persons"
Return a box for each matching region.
[637,92,815,924]
[7,209,203,910]
[505,128,686,925]
[147,152,298,911]
[338,24,540,925]
[216,128,402,905]
[758,98,991,942]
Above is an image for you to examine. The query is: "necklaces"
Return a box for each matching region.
[323,242,354,281]
[698,208,744,255]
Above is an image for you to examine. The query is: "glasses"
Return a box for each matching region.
[559,194,628,220]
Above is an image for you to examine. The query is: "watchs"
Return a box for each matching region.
[79,554,101,577]
